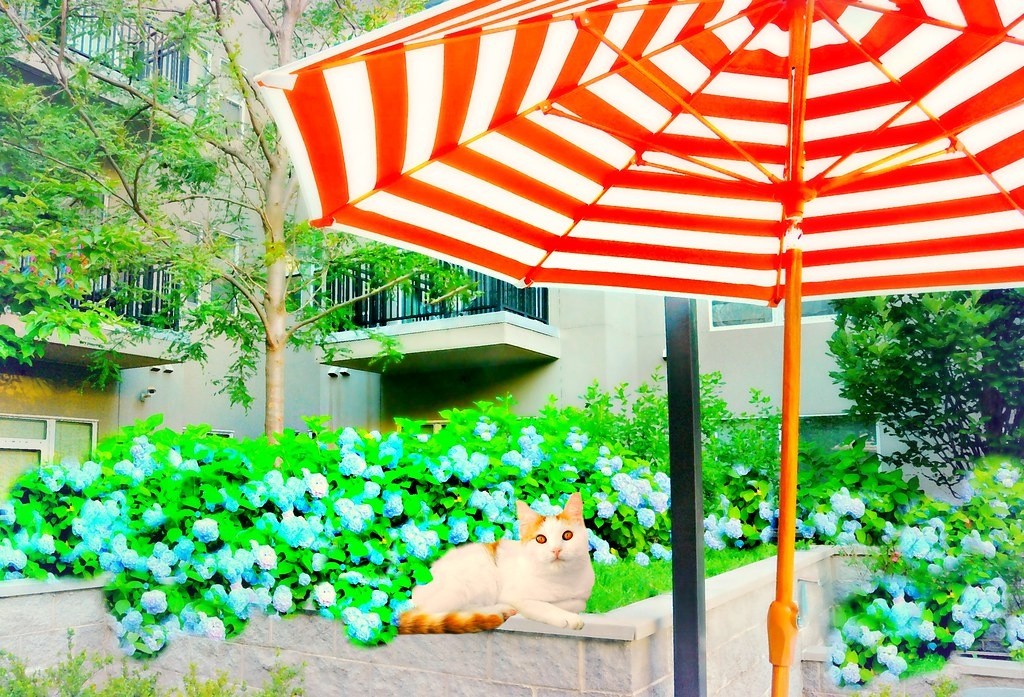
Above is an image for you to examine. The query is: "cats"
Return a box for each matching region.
[396,489,597,636]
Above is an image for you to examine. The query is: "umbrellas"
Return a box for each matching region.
[254,0,1024,697]
